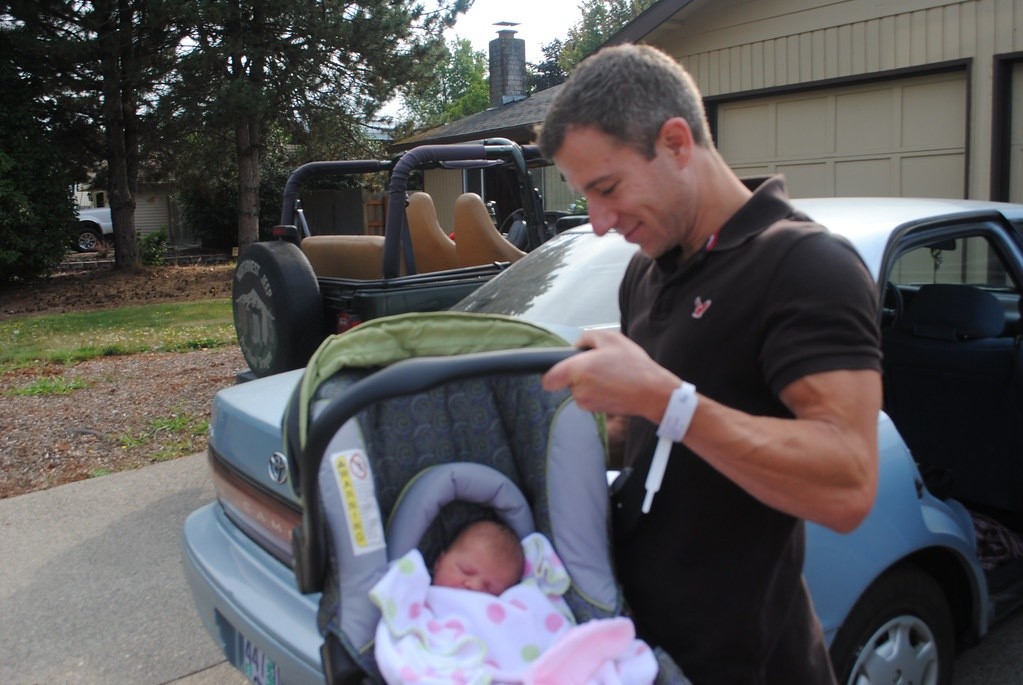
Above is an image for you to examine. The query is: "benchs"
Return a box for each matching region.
[300,235,386,280]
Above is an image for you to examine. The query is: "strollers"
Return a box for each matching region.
[279,309,694,685]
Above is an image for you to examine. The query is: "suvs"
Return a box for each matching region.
[232,138,590,385]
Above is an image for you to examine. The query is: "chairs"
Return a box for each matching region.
[883,284,1023,512]
[452,193,527,267]
[406,192,455,273]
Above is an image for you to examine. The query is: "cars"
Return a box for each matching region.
[70,207,114,252]
[180,197,1023,685]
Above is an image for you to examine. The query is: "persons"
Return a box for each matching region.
[536,45,882,685]
[428,516,524,597]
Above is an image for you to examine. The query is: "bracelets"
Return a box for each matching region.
[640,381,700,514]
